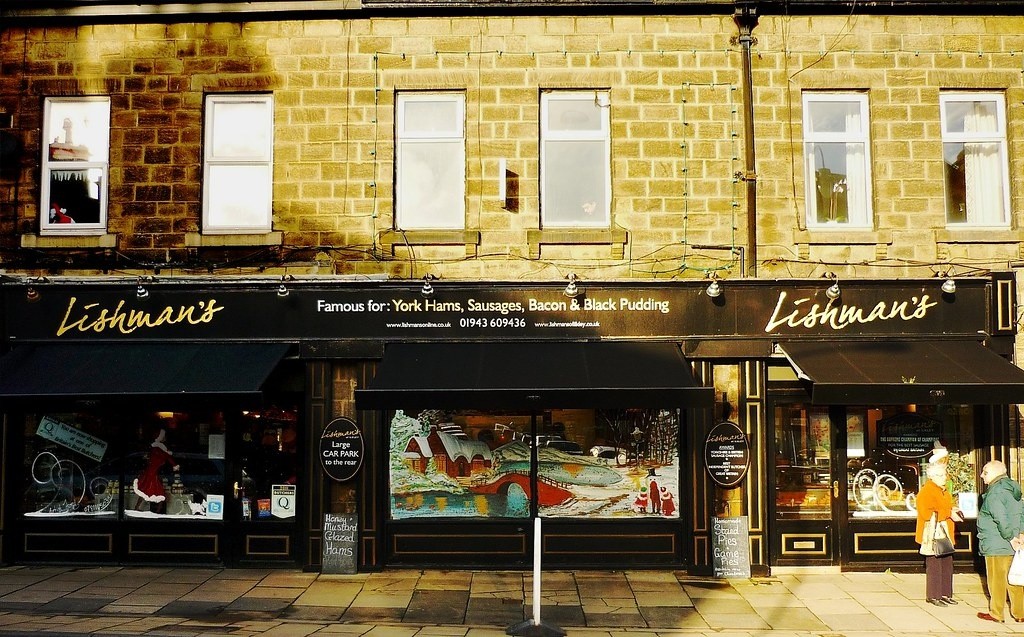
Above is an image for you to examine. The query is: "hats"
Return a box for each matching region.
[152,429,166,442]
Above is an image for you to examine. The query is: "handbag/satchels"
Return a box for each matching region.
[932,521,956,558]
[1007,543,1024,586]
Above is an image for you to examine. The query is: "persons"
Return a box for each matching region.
[134,427,180,514]
[914,463,963,607]
[977,461,1024,624]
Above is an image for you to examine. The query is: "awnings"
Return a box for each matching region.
[354,343,716,408]
[779,340,1023,404]
[0,340,295,396]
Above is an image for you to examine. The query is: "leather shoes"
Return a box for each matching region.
[1010,613,1024,622]
[977,613,1004,622]
[940,595,958,604]
[926,595,947,607]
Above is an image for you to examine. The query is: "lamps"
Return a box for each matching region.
[420,271,435,298]
[136,275,154,302]
[706,270,721,298]
[26,275,46,303]
[938,266,956,293]
[276,273,294,300]
[563,272,580,298]
[825,271,841,301]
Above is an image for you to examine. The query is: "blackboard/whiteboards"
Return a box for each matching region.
[322,513,358,575]
[711,515,750,580]
